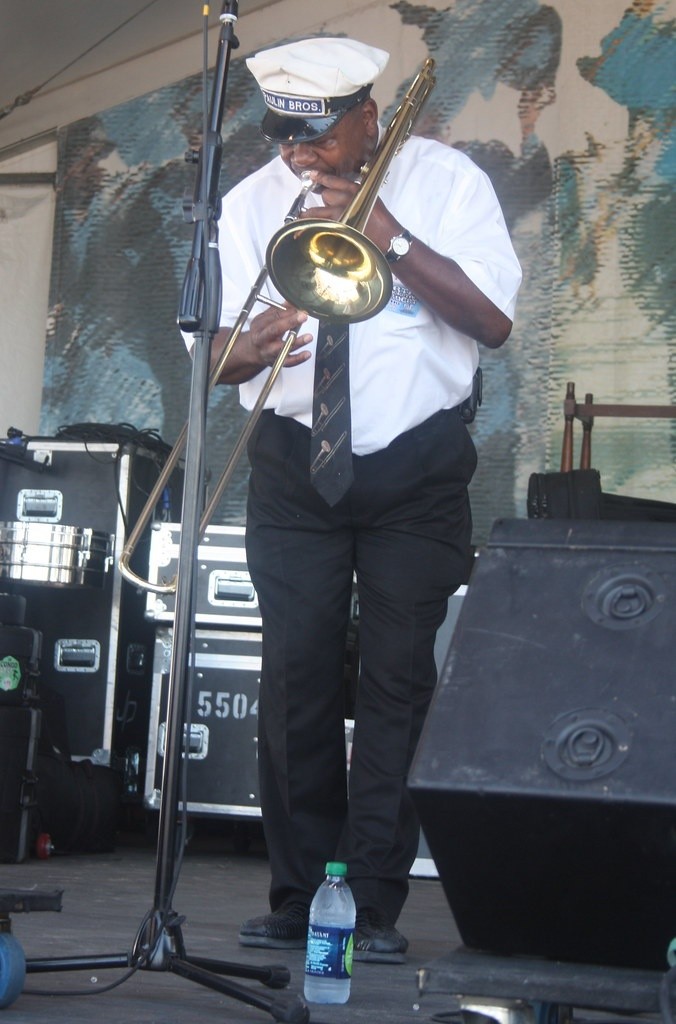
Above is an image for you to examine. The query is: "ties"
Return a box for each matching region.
[308,318,355,506]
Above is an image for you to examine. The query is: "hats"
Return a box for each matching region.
[245,35,392,145]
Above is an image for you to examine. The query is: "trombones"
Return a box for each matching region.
[113,55,439,596]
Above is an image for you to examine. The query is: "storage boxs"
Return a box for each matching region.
[0,438,469,880]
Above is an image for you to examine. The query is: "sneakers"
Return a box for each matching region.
[238,904,312,951]
[350,912,411,964]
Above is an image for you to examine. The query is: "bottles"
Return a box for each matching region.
[304,861,356,1004]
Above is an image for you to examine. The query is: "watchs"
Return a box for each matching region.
[383,228,414,265]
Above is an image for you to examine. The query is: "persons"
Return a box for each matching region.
[181,38,526,964]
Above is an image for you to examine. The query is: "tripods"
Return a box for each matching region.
[24,1,311,1024]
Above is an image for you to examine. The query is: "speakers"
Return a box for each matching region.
[410,516,676,962]
[0,594,48,863]
